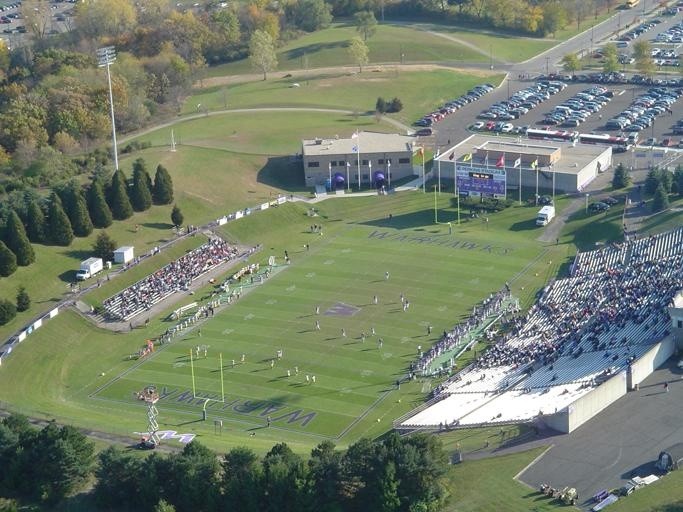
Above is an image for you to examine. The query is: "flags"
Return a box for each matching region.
[412,144,557,171]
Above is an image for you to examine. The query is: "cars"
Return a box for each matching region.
[414,76,614,135]
[590,0,683,66]
[588,197,618,212]
[604,87,683,131]
[647,119,683,149]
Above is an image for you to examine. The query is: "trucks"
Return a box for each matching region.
[75,257,103,281]
[535,205,555,227]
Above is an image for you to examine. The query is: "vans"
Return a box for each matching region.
[628,132,638,144]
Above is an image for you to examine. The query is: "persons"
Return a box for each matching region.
[73,192,683,449]
